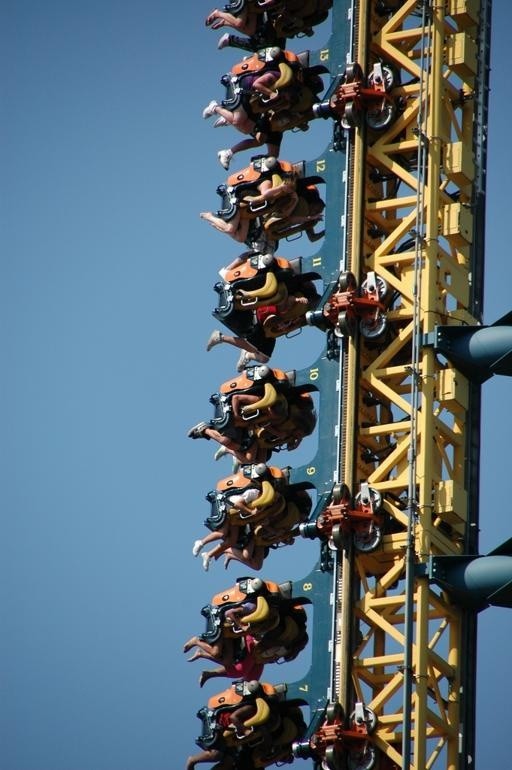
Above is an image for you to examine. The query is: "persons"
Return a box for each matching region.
[183,0,330,770]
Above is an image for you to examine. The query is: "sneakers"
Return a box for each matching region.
[201,552,211,571]
[207,330,223,352]
[237,349,251,373]
[215,445,226,461]
[213,115,232,128]
[232,456,241,474]
[187,422,208,438]
[218,150,231,171]
[200,671,211,688]
[206,10,230,49]
[192,540,204,557]
[202,100,219,120]
